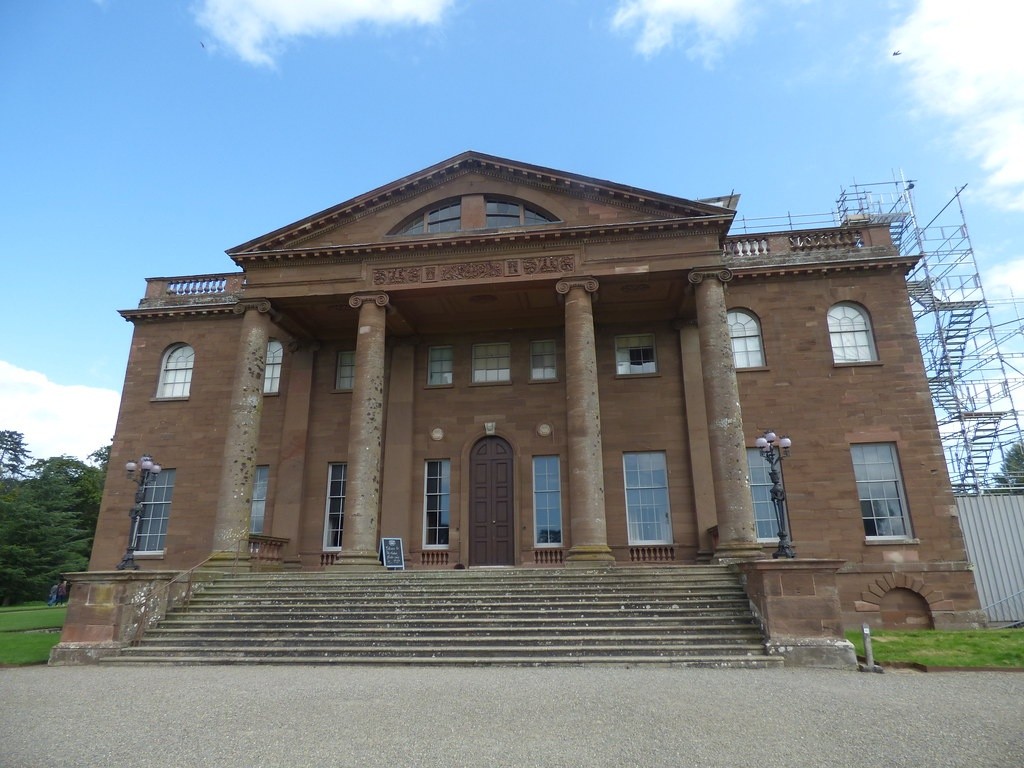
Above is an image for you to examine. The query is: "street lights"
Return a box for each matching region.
[755,427,799,558]
[115,452,162,570]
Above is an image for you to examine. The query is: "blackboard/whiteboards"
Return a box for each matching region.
[378,538,405,567]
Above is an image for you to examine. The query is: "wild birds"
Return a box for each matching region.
[200,40,206,49]
[892,51,901,56]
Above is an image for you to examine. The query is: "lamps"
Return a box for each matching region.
[125,453,161,484]
[756,430,791,465]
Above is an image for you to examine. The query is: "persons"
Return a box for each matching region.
[55,581,67,606]
[48,583,59,606]
